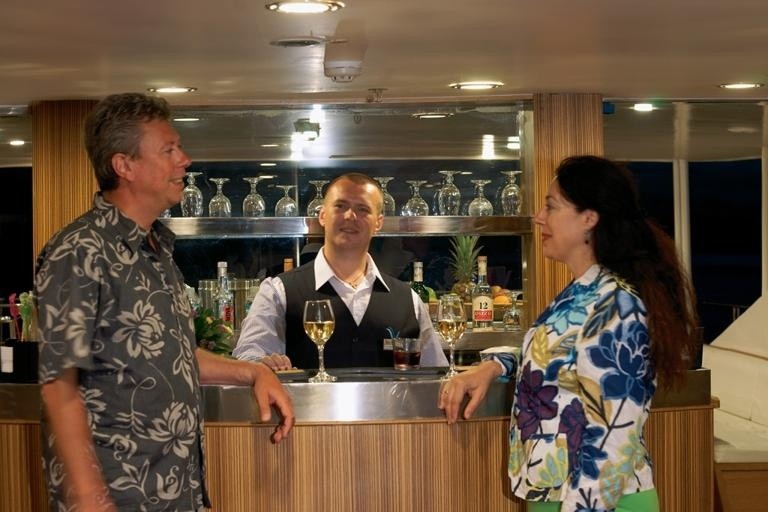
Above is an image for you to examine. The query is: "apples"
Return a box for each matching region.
[425,286,436,300]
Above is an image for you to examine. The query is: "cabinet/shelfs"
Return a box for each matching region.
[160,215,531,350]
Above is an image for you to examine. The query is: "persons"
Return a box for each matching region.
[438,155,700,512]
[34,92,295,512]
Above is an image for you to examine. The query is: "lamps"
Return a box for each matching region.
[448,80,504,89]
[265,0,346,13]
[412,113,454,119]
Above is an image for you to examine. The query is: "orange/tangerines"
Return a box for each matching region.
[491,285,512,304]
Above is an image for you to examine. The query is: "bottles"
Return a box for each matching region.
[211,261,234,329]
[283,257,294,273]
[410,260,430,313]
[470,254,495,329]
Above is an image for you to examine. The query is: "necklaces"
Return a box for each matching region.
[333,271,365,290]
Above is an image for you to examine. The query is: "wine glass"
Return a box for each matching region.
[372,176,398,216]
[301,300,338,385]
[407,179,432,217]
[497,169,523,217]
[157,168,267,218]
[434,296,465,380]
[501,289,521,332]
[307,179,330,217]
[436,169,461,216]
[272,183,300,217]
[467,178,494,217]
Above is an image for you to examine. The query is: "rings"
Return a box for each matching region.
[444,389,448,394]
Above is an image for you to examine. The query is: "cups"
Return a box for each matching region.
[392,337,422,373]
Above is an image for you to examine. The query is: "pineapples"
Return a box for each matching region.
[446,236,486,304]
